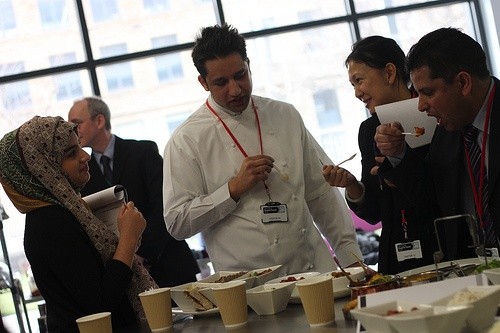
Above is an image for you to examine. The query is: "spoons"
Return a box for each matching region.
[334,153,356,166]
[401,132,421,136]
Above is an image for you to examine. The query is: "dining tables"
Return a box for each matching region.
[142,258,500,333]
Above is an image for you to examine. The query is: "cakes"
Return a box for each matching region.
[183,287,218,311]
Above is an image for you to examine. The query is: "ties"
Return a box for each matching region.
[460,127,498,249]
[99,155,112,186]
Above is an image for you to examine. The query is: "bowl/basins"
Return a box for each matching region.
[319,267,366,291]
[246,282,296,315]
[349,301,432,333]
[264,272,321,296]
[430,285,500,333]
[482,268,500,285]
[346,275,401,299]
[170,282,221,312]
[197,271,256,290]
[251,265,282,286]
[385,305,475,333]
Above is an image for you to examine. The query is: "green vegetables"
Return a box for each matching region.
[472,258,500,276]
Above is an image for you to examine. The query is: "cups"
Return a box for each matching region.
[76,312,113,333]
[295,276,335,327]
[138,288,173,333]
[210,281,248,328]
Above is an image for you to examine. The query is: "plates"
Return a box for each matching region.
[171,304,220,315]
[291,287,353,300]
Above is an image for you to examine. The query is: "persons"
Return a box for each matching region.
[162,25,378,288]
[373,26,500,259]
[0,114,160,332]
[320,34,439,273]
[68,98,202,289]
